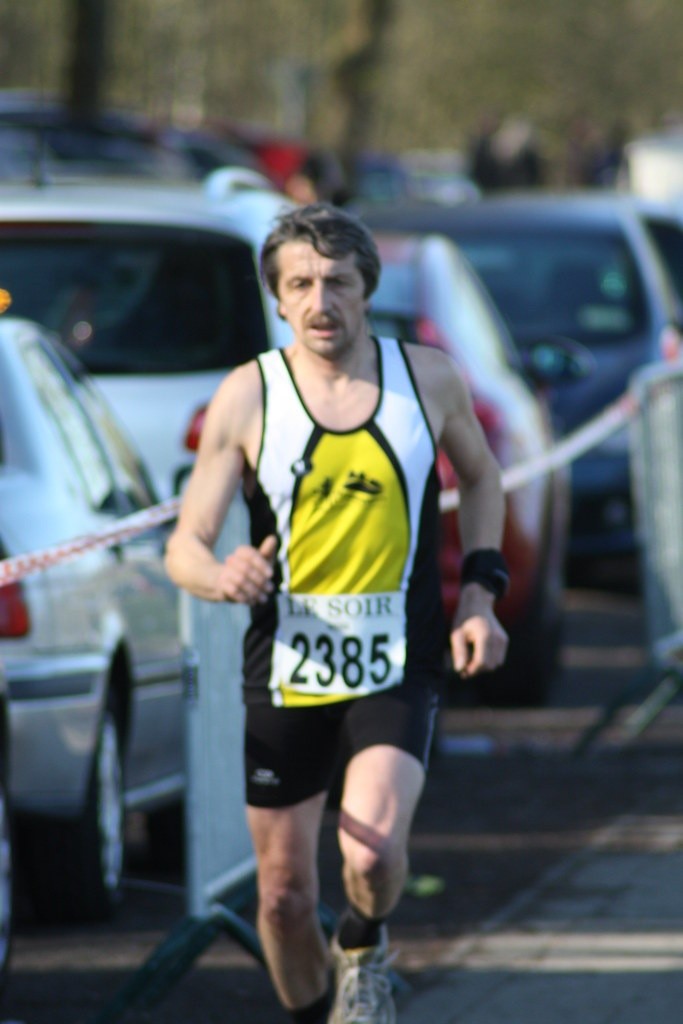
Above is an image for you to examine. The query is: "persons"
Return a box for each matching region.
[164,203,508,1024]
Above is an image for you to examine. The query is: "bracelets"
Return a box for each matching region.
[461,547,505,599]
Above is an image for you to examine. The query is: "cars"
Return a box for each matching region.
[0,289,191,914]
[0,100,682,711]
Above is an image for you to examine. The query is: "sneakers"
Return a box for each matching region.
[328,924,400,1024]
